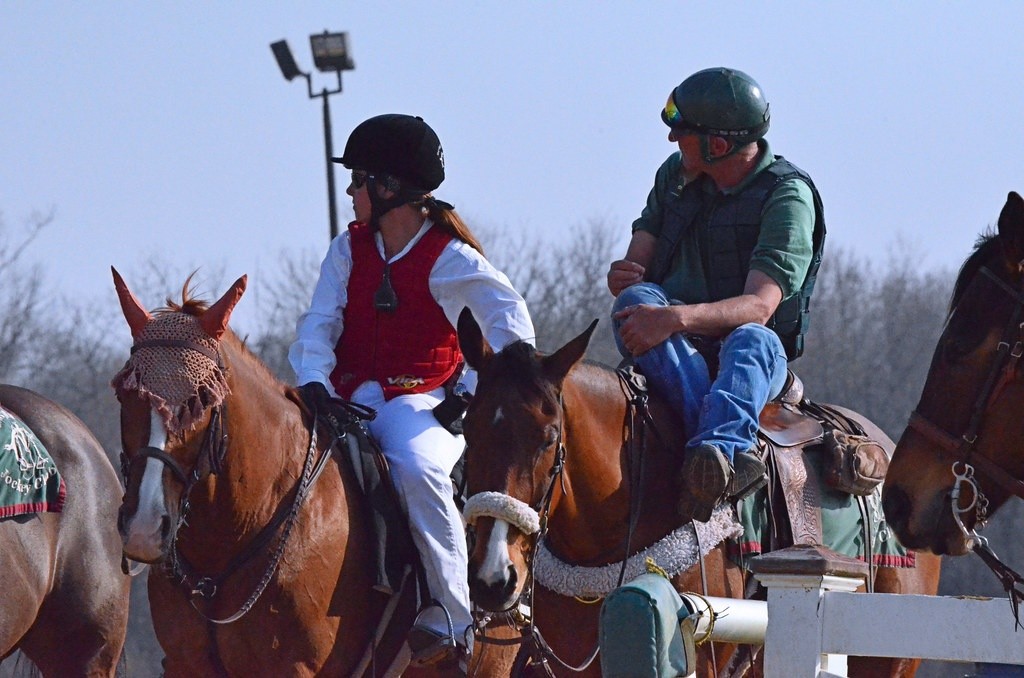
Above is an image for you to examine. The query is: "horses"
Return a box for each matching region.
[0,380,135,677]
[109,263,944,677]
[878,188,1023,561]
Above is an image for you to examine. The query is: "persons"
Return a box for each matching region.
[607,67,828,523]
[288,115,538,678]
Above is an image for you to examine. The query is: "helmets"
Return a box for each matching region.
[660,67,771,164]
[329,114,446,194]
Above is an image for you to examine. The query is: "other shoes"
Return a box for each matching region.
[408,622,474,661]
[679,444,770,523]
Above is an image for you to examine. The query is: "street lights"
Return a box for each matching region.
[270,29,355,242]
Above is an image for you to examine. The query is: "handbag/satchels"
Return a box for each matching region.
[823,428,890,497]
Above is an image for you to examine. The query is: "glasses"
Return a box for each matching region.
[665,87,687,126]
[350,173,374,188]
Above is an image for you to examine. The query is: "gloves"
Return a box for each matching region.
[432,394,468,434]
[296,381,331,415]
